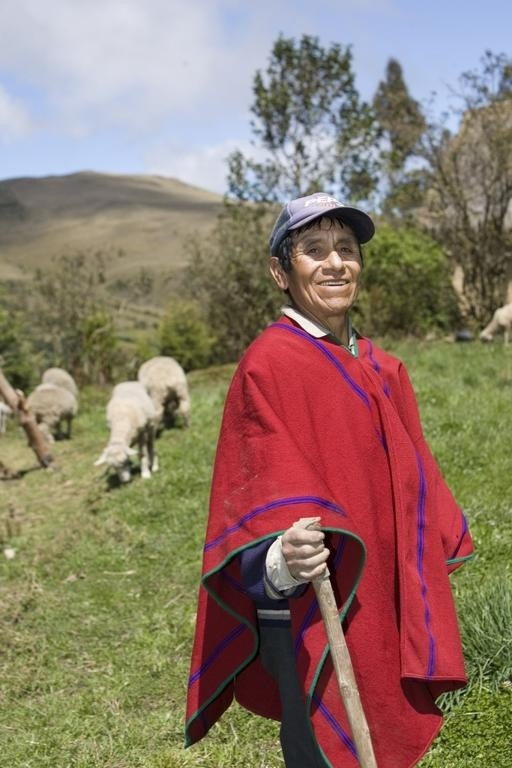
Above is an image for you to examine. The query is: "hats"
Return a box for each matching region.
[268,192,374,257]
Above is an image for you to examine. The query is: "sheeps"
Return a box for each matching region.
[479,304,512,344]
[94,379,160,485]
[136,356,193,433]
[22,384,79,444]
[0,389,25,433]
[40,367,80,397]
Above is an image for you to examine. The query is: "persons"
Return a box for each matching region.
[183,190,477,767]
